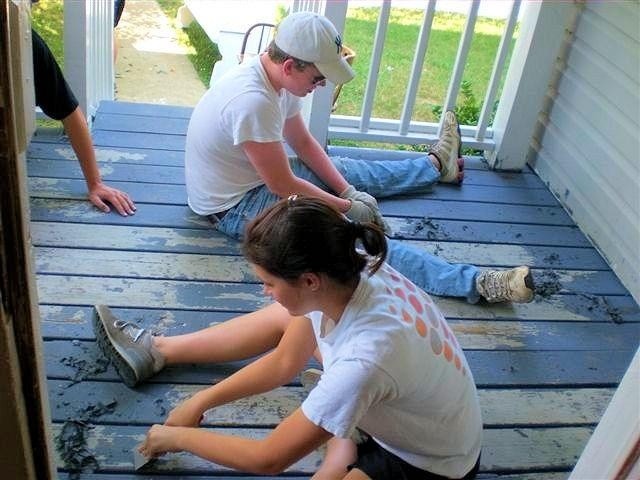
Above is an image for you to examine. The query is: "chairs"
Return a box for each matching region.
[237,25,354,113]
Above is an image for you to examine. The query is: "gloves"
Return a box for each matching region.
[341,183,386,228]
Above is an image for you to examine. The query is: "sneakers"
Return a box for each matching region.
[428,111,465,185]
[477,264,535,304]
[90,304,169,387]
[299,369,369,446]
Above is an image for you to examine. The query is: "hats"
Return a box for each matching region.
[273,12,356,84]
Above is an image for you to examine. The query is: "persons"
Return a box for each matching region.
[114,0,124,90]
[185,10,533,304]
[92,194,482,480]
[32,29,136,217]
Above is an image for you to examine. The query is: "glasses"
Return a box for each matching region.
[297,60,325,88]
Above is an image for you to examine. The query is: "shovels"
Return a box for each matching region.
[369,223,398,238]
[134,411,206,471]
